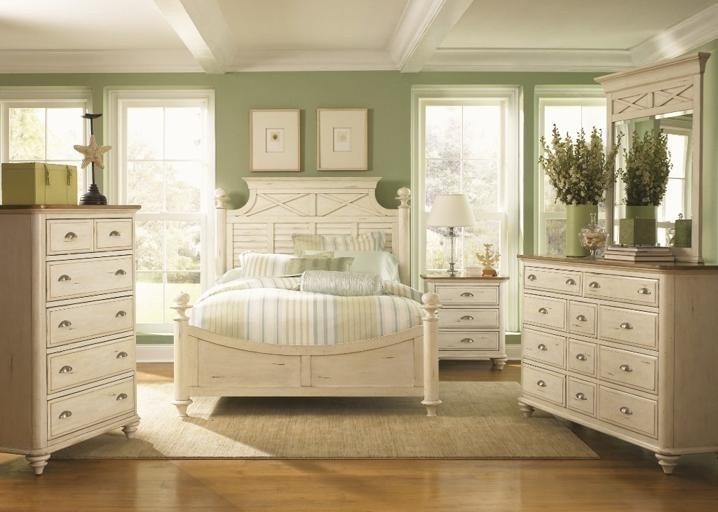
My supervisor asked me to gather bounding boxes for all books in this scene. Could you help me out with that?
[603,244,674,266]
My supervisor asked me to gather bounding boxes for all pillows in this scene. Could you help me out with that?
[240,251,399,296]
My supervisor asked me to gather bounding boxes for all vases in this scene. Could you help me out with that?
[589,246,605,261]
[626,204,655,218]
[566,204,598,256]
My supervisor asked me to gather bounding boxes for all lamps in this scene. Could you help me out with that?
[428,192,476,272]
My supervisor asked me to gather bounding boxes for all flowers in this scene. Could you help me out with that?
[621,128,672,206]
[580,224,608,249]
[535,124,625,205]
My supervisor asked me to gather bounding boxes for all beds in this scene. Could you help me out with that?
[170,177,442,420]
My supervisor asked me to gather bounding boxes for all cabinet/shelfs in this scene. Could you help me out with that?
[518,255,718,474]
[0,205,141,475]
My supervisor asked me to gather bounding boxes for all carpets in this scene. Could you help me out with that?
[25,381,601,460]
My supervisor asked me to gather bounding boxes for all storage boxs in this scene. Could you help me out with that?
[2,163,77,205]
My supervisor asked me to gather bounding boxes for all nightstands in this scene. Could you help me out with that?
[420,269,510,371]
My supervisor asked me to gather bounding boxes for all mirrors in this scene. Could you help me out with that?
[594,52,711,263]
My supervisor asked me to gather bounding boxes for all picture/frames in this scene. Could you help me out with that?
[250,109,300,171]
[317,108,368,171]
